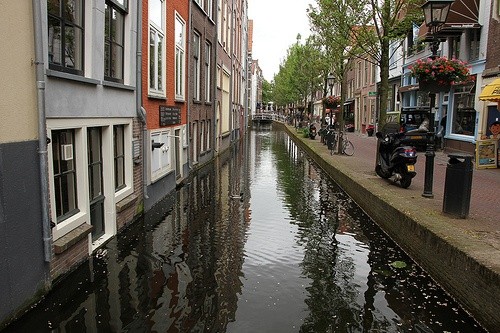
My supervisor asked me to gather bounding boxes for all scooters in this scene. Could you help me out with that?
[308,122,317,139]
[375,131,418,189]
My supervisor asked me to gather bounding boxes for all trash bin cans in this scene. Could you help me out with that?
[327,125,337,150]
[442,153,475,219]
[288,116,291,123]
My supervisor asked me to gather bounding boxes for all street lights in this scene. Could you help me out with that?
[327,72,337,149]
[422,0,454,197]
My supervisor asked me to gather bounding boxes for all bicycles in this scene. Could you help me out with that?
[321,124,355,156]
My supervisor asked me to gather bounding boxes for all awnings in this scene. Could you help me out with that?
[478,78,500,101]
